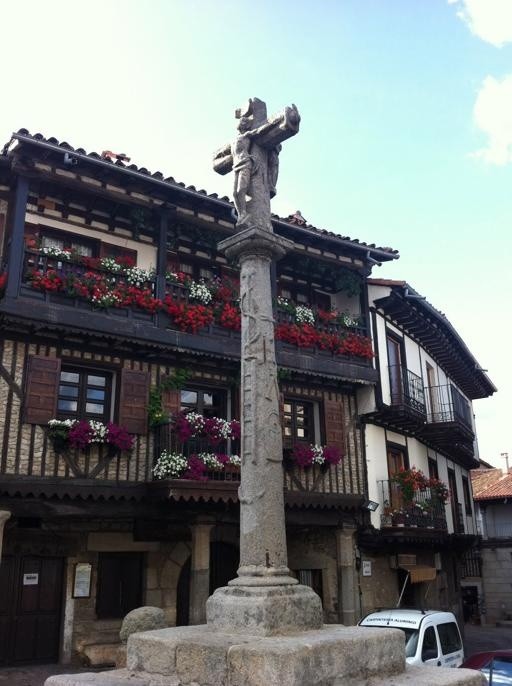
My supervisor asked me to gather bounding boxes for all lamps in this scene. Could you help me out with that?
[362,499,379,511]
[14,515,42,531]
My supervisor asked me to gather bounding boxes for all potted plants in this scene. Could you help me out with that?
[380,500,392,528]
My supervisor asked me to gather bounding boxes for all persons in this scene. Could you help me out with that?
[212,114,285,225]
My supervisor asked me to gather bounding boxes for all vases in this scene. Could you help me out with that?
[397,524,404,527]
[410,525,418,528]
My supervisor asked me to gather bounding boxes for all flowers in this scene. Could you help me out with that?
[390,465,449,524]
[151,411,343,481]
[23,232,378,359]
[47,418,134,451]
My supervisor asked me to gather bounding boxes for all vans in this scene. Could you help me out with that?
[355,606,467,669]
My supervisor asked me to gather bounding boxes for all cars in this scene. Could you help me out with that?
[458,648,512,686]
